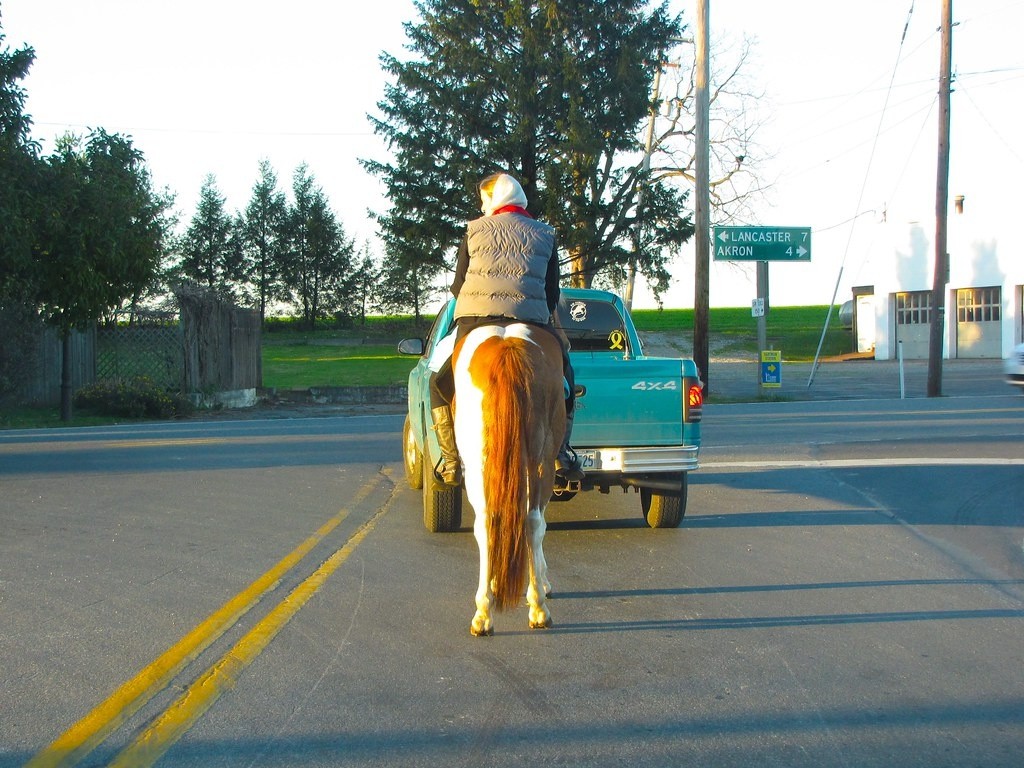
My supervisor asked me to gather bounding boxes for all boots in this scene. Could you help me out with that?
[430,405,464,486]
[554,418,585,482]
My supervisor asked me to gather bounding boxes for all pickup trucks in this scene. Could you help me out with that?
[394,287,705,533]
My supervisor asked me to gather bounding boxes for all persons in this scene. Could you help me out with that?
[428,173,585,492]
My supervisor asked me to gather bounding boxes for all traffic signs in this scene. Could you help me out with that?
[712,226,813,262]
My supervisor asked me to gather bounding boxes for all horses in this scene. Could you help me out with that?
[447,319,567,638]
